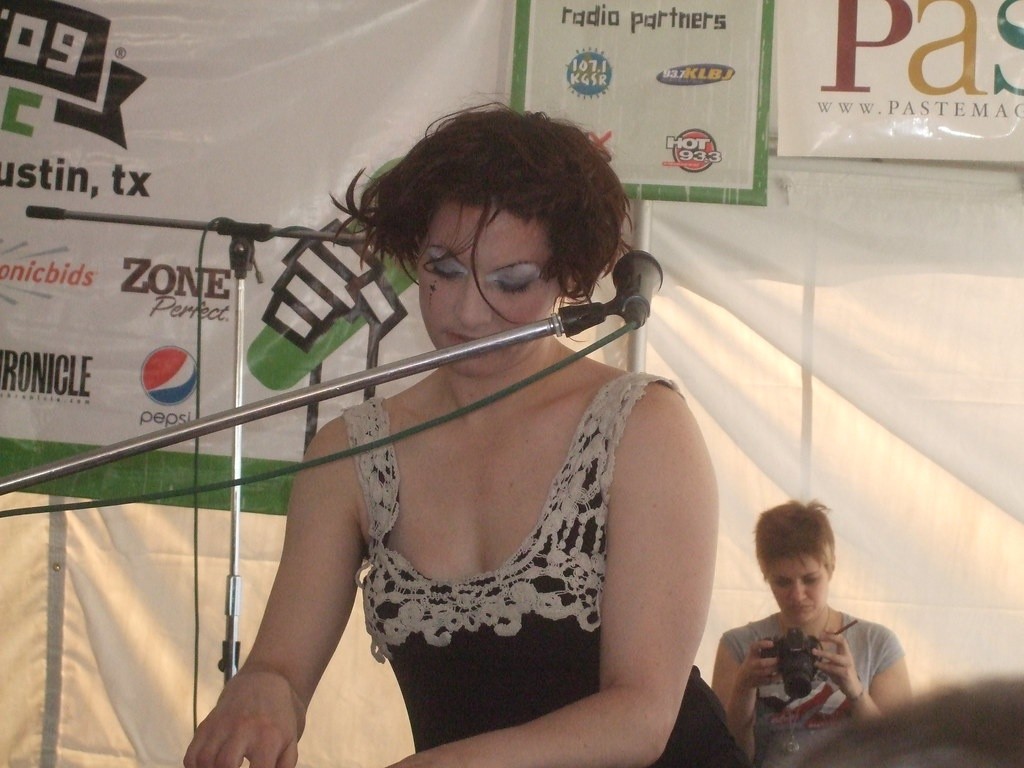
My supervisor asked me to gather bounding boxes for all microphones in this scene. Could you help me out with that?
[613,250,663,330]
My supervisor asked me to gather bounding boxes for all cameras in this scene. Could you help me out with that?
[761,627,824,698]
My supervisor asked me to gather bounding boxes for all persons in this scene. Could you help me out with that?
[712,499,910,767]
[182,101,719,768]
